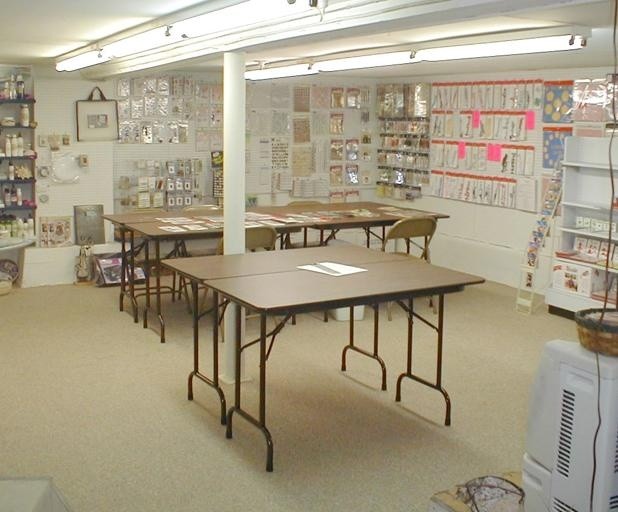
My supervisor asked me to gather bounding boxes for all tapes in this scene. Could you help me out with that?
[40,194,48,203]
[39,167,49,178]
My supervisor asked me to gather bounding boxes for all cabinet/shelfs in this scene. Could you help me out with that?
[545,136,618,320]
[0,98,39,251]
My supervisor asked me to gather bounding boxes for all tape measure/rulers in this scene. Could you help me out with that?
[314,263,340,274]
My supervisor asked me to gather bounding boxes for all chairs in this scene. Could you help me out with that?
[198,225,276,344]
[380,214,438,321]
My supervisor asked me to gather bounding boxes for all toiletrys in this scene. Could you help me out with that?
[8,160,15,180]
[5,132,23,156]
[0,216,34,241]
[4,73,25,99]
[4,183,22,206]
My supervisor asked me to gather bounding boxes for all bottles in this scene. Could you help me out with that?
[2,117,15,126]
[0,73,26,99]
[19,104,31,127]
[9,162,15,180]
[4,183,23,207]
[6,132,23,157]
[0,213,34,240]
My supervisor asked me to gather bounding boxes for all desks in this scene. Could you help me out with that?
[203,258,485,473]
[159,243,412,425]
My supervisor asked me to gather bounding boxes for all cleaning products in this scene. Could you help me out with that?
[0,71,36,243]
